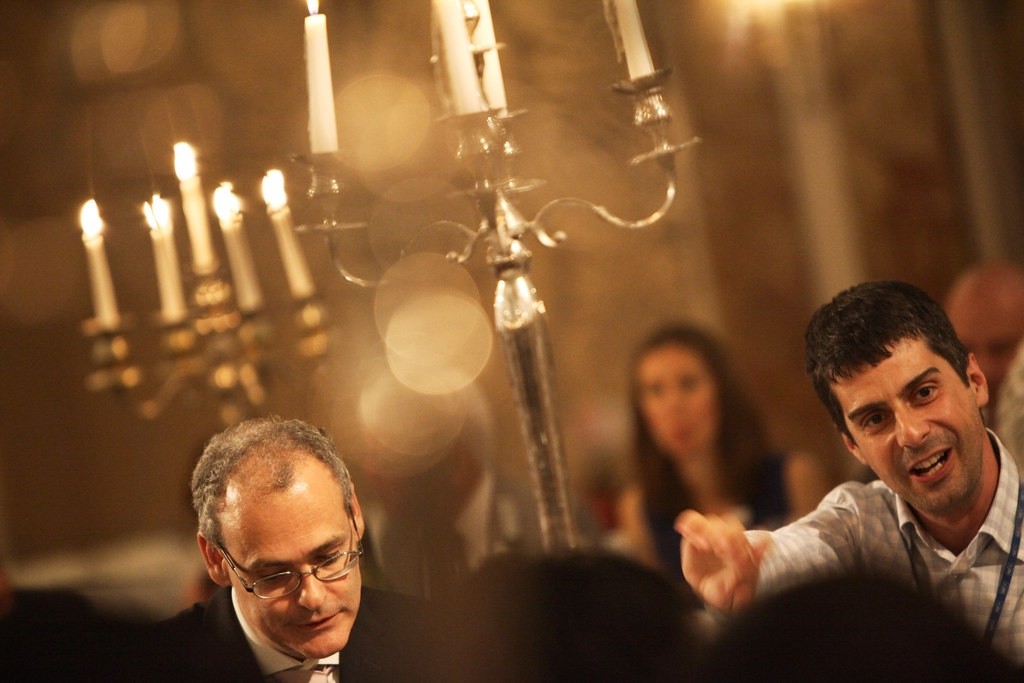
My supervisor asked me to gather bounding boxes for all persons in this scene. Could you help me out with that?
[624,318,830,597]
[140,416,486,683]
[681,279,1022,672]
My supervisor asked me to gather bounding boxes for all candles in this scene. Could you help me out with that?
[602,2,655,81]
[301,0,344,154]
[172,141,221,277]
[211,182,265,316]
[261,168,318,301]
[430,0,508,122]
[142,193,186,324]
[78,197,123,331]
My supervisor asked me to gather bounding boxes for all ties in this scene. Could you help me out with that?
[271,664,335,683]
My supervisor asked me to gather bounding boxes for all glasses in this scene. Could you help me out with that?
[217,502,363,598]
